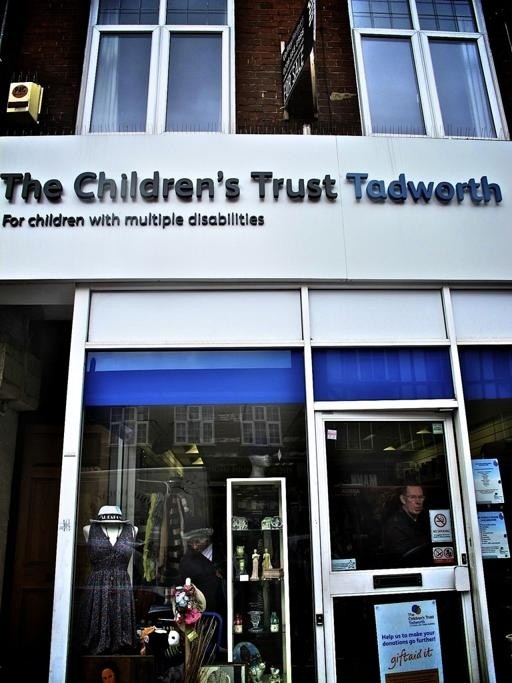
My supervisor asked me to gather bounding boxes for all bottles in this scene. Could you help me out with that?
[233,612,243,634]
[270,610,280,633]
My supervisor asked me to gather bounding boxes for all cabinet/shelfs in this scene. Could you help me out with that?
[226,477,292,683]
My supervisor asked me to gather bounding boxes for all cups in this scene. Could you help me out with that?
[248,610,264,631]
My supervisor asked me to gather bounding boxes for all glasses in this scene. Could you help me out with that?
[404,495,426,501]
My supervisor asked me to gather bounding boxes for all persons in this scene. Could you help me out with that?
[383,482,455,570]
[95,660,123,682]
[245,454,273,477]
[82,522,140,655]
[252,548,261,579]
[262,548,274,581]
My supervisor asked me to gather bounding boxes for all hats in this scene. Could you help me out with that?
[90,505,130,523]
[183,518,212,540]
